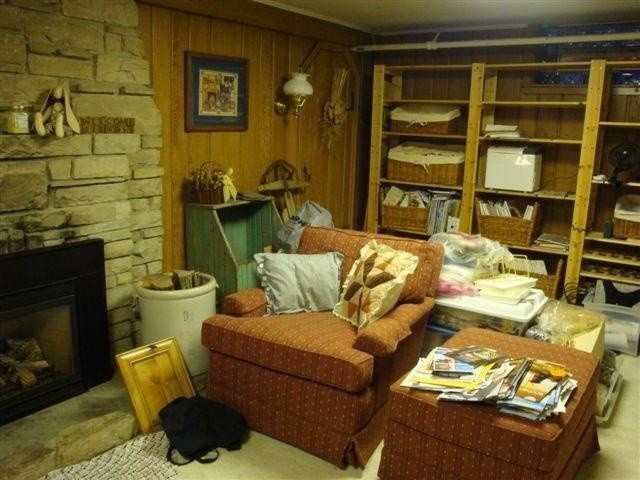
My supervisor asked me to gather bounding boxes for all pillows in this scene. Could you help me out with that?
[253,252,344,316]
[332,238,421,334]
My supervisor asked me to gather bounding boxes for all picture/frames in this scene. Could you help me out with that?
[184,50,248,132]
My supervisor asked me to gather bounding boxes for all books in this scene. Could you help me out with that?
[483,124,518,132]
[479,288,530,305]
[534,232,568,252]
[475,196,533,220]
[473,271,539,291]
[485,132,522,138]
[382,184,462,236]
[399,344,579,423]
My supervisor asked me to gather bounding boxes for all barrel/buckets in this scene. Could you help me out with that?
[136,271,216,378]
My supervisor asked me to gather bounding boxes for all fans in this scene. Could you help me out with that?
[606,144,639,183]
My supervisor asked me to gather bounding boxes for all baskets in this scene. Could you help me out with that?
[610,193,640,240]
[473,196,545,247]
[379,102,467,232]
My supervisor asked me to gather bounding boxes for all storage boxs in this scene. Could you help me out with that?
[421,288,549,359]
[485,145,544,192]
[582,289,640,357]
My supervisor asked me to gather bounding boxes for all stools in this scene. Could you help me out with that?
[377,326,601,479]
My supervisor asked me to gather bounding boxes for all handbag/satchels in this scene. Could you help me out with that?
[158,394,249,465]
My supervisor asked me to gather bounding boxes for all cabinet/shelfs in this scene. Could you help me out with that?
[366,59,640,304]
[184,192,284,313]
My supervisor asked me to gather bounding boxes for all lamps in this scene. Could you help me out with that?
[274,65,314,118]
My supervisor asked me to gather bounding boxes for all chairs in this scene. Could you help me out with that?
[202,226,444,467]
